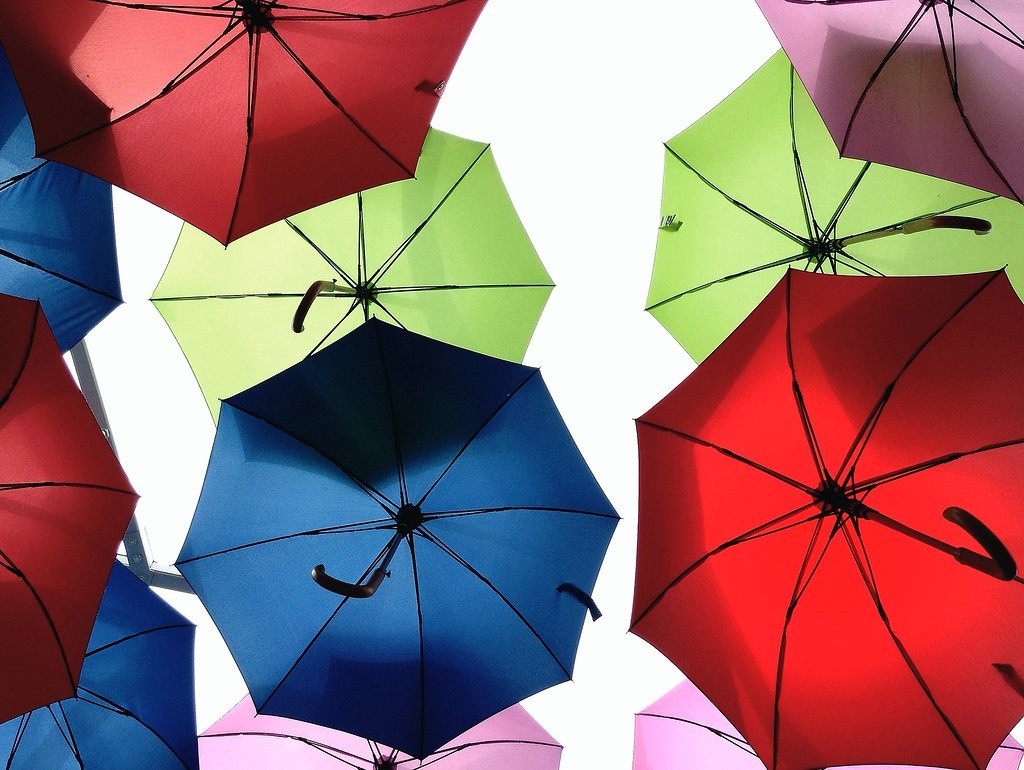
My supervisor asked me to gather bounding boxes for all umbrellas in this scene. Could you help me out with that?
[642,45,1022,368]
[622,271,1024,768]
[755,0,1020,203]
[0,0,616,770]
